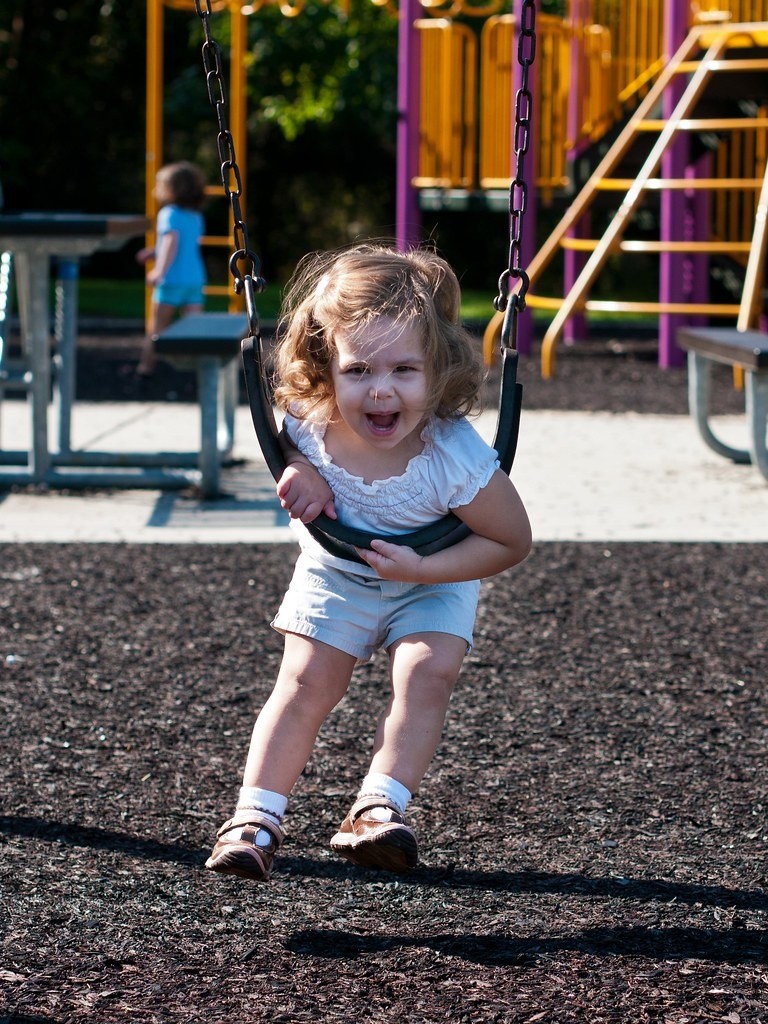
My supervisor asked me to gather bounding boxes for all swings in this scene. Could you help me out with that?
[190,1,536,562]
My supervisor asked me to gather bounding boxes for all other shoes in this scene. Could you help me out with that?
[115,366,150,391]
[204,814,284,876]
[329,793,419,866]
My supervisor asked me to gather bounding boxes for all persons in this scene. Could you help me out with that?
[199,244,534,868]
[139,162,205,375]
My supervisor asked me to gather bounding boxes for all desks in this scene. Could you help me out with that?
[0,212,225,491]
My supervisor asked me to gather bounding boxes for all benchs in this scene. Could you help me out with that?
[674,324,768,488]
[151,310,255,503]
